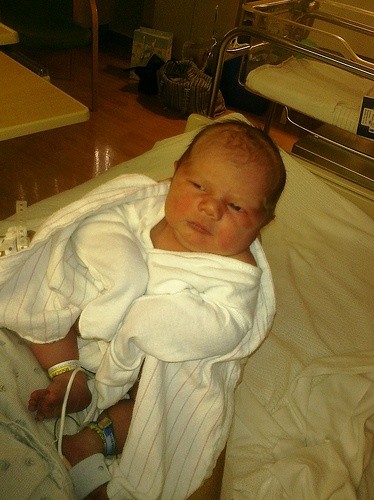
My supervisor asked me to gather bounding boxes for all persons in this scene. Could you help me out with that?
[25,120,287,500]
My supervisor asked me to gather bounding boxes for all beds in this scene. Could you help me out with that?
[238,0,374,161]
[0,114,374,500]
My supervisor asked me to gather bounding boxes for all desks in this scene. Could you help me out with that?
[0,51,91,145]
